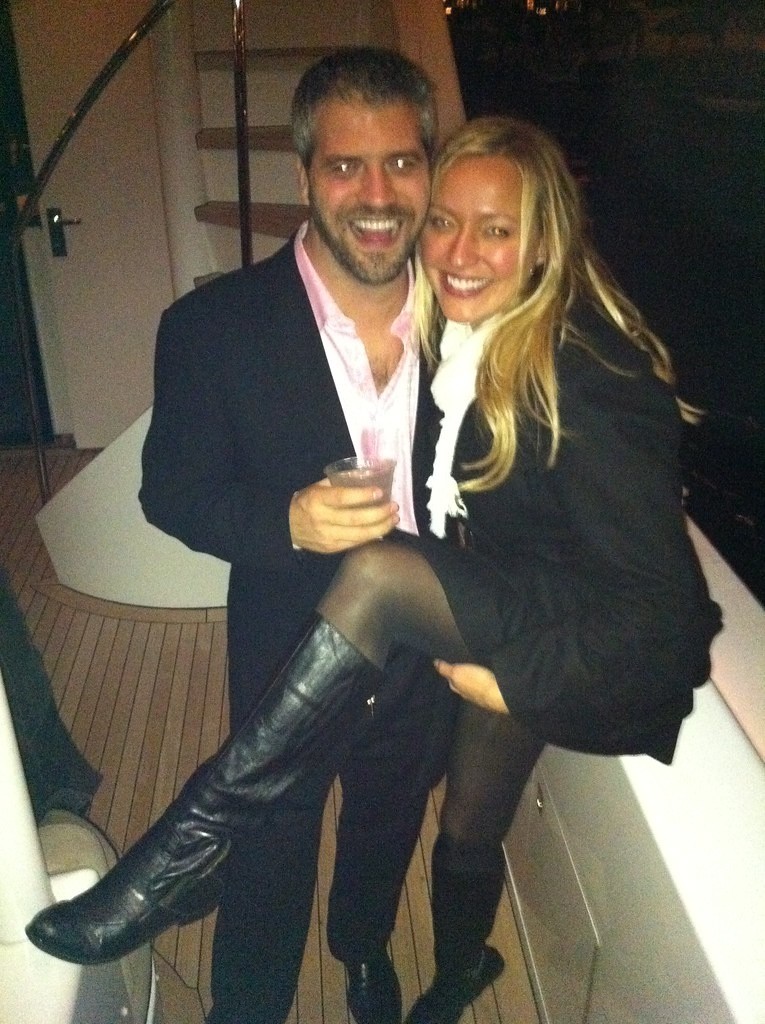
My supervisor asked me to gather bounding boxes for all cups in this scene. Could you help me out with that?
[324,456,398,542]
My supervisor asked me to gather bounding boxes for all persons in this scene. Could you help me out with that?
[1,560,103,830]
[26,116,724,1024]
[138,46,457,1024]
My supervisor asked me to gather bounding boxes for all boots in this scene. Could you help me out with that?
[21,606,386,964]
[396,840,508,1023]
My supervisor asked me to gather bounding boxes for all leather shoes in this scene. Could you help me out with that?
[337,959,401,1024]
